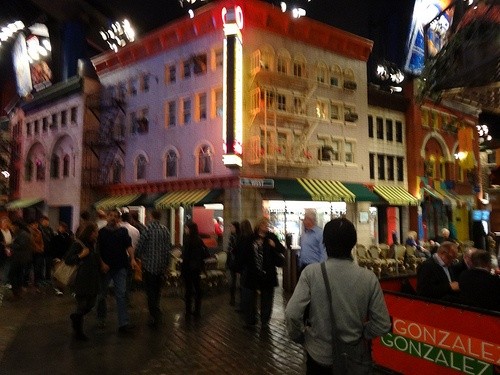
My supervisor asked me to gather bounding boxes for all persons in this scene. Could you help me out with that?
[0,206,500,375]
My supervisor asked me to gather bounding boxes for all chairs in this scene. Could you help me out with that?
[357,242,431,280]
[168,248,226,296]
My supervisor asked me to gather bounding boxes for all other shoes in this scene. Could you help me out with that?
[118,323,136,331]
[96,320,108,328]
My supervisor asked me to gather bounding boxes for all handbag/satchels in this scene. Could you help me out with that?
[52,239,88,288]
[332,335,374,375]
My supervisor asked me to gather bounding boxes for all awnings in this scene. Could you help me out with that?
[423,187,466,206]
[258,176,379,203]
[8,198,43,208]
[91,188,222,209]
[373,185,420,206]
